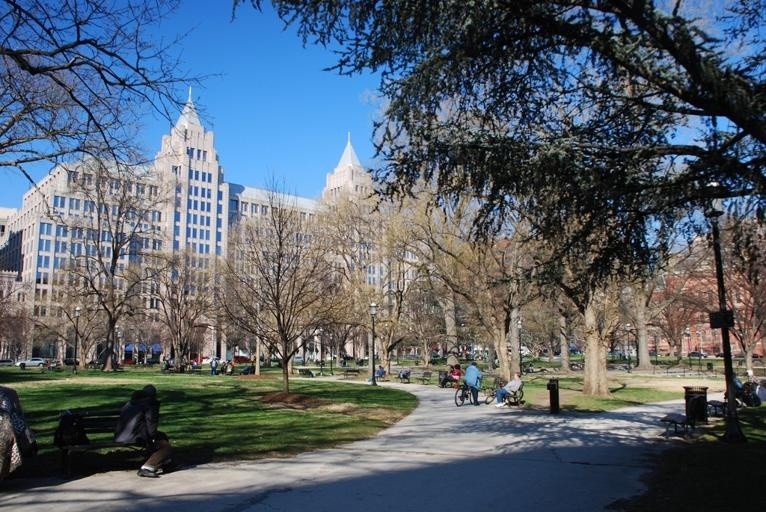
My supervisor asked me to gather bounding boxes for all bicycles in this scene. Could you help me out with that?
[455,381,474,406]
[485,388,523,405]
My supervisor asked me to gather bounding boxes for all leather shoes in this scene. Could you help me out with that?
[137,468,166,478]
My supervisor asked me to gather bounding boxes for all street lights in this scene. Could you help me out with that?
[517,320,524,376]
[73,307,80,374]
[114,325,118,371]
[369,303,379,385]
[703,182,748,444]
[625,323,632,373]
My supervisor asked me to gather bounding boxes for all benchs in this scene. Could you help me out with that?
[660,392,728,440]
[416,372,431,384]
[21,404,138,479]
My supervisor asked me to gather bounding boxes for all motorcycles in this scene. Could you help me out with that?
[734,373,761,408]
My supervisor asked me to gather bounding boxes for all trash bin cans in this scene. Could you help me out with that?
[683,386,708,424]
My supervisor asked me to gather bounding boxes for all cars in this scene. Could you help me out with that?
[17,358,47,369]
[0,359,14,367]
[688,351,707,357]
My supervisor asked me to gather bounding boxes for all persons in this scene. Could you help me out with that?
[113,383,170,477]
[374,364,385,376]
[439,364,462,387]
[210,358,218,375]
[496,372,522,406]
[465,362,483,405]
[746,369,760,386]
[724,371,744,398]
[160,355,199,374]
[449,366,455,374]
[220,361,227,375]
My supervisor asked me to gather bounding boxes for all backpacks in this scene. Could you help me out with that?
[54,408,92,448]
